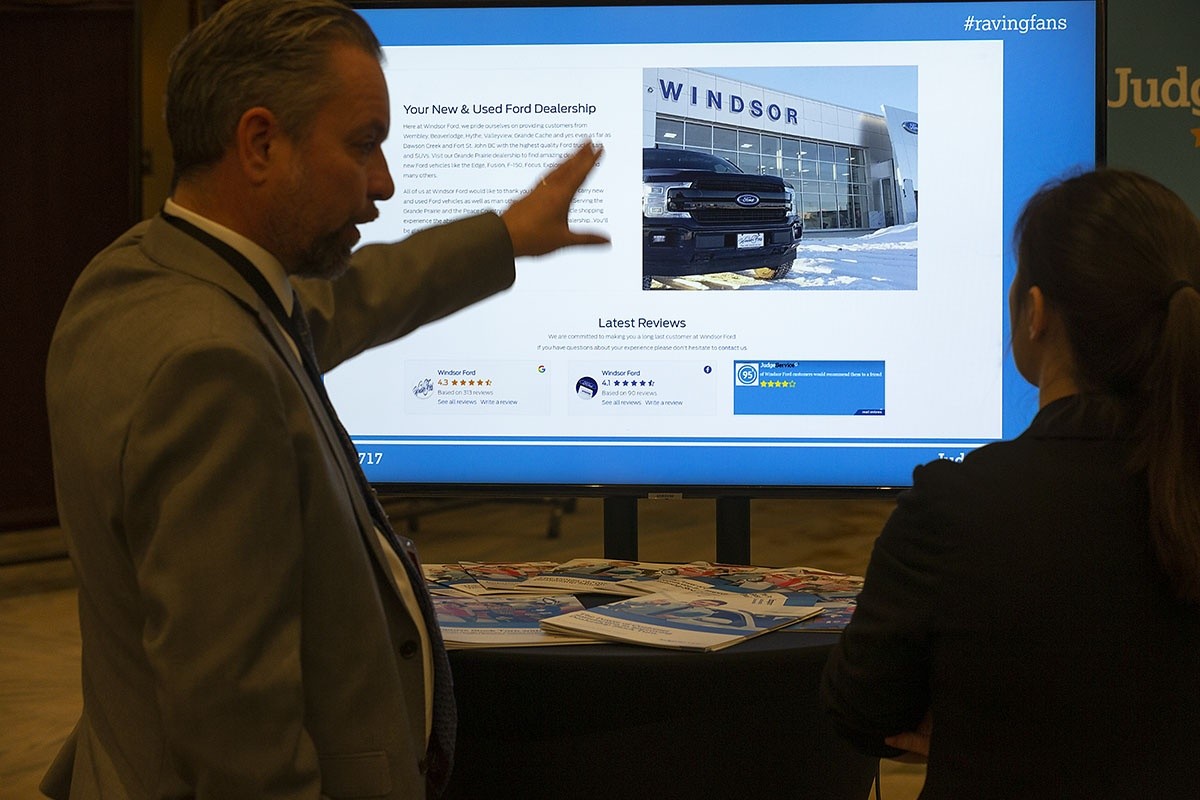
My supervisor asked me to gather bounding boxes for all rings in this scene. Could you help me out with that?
[542,180,547,186]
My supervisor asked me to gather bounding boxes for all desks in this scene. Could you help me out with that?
[420,559,864,800]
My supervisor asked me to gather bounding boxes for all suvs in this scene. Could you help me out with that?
[641,146,804,293]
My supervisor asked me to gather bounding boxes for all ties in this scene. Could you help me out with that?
[291,289,456,795]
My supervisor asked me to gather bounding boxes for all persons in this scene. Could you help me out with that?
[619,598,727,621]
[824,169,1200,800]
[41,0,611,800]
